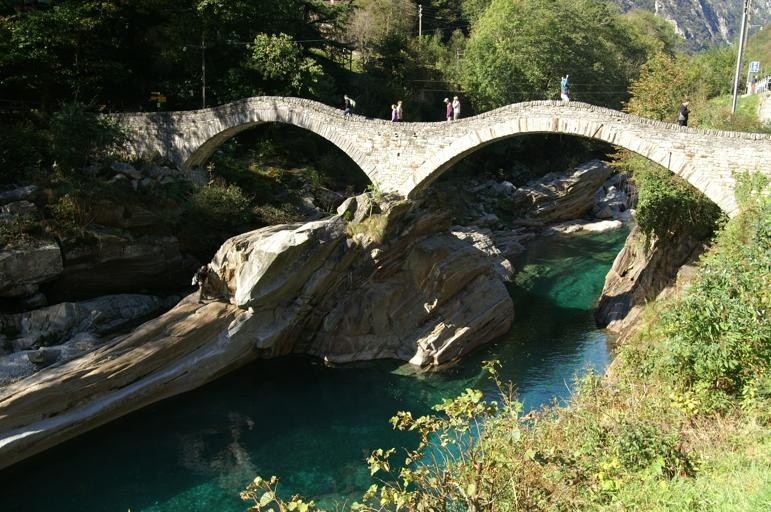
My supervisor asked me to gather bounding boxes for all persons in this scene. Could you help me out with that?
[196,265,212,304]
[398,100,404,122]
[344,95,354,117]
[443,98,454,120]
[679,99,692,126]
[562,75,571,102]
[452,96,461,120]
[391,104,398,122]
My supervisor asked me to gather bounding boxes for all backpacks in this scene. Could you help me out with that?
[349,98,355,108]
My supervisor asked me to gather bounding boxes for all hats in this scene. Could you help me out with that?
[443,96,458,102]
